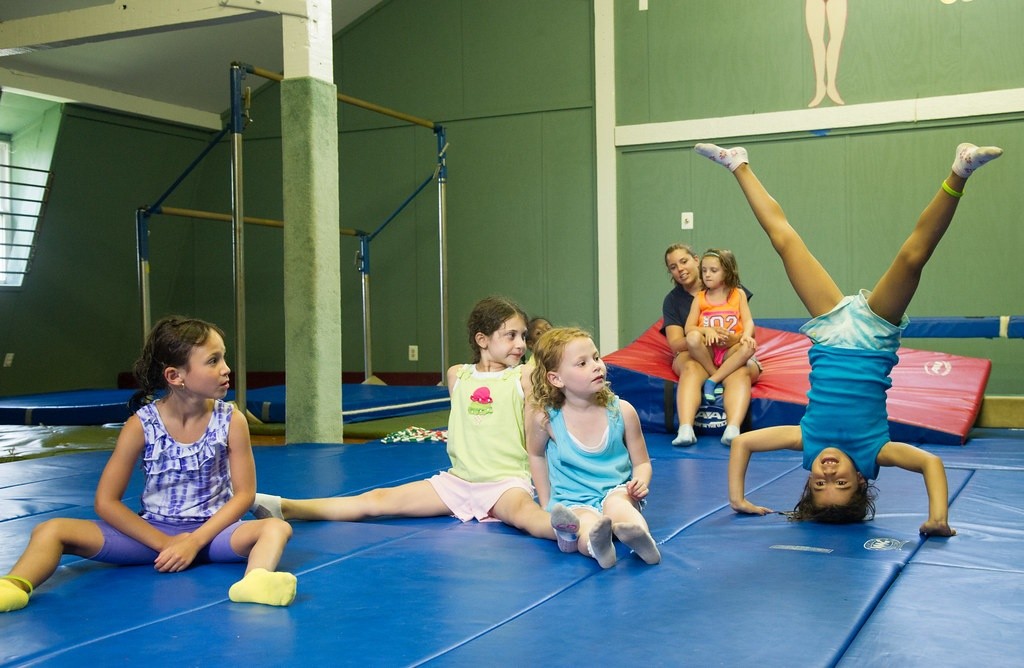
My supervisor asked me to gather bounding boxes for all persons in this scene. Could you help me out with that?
[659,243,763,446]
[1,313,298,612]
[694,139,998,537]
[525,316,556,366]
[527,327,661,570]
[248,294,581,553]
